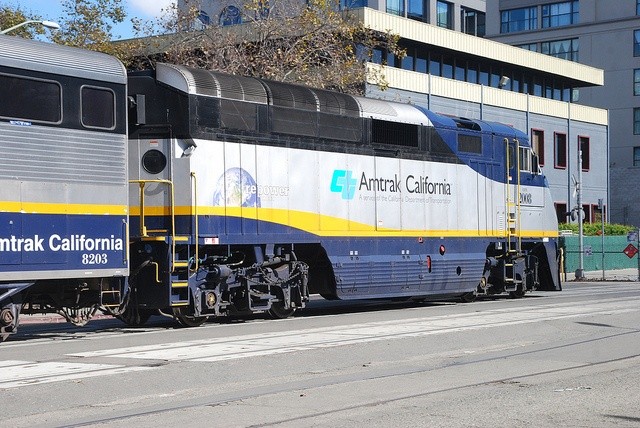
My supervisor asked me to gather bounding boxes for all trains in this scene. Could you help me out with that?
[0,33,565,342]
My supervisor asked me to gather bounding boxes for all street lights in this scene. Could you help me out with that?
[1,20,60,34]
